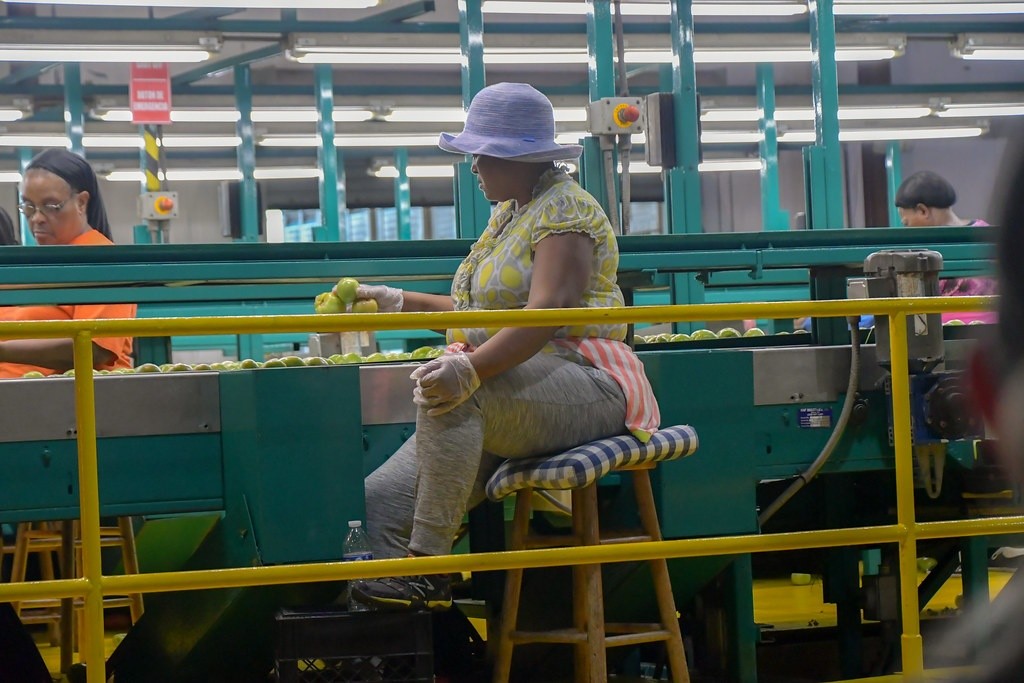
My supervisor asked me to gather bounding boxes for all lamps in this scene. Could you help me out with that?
[0,89,1024,184]
[949,32,1024,61]
[283,32,909,64]
[0,29,224,63]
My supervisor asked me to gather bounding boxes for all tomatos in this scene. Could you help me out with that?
[632,328,765,343]
[941,319,985,327]
[20,278,443,379]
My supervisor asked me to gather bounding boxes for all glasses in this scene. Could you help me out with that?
[17,191,77,215]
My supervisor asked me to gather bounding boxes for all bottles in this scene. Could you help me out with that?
[343,520,377,612]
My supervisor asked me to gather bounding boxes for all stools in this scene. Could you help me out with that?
[10,516,145,674]
[492,460,690,683]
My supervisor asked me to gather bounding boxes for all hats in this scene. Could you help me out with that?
[438,81,581,164]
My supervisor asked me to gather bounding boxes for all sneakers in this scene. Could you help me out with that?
[351,552,453,612]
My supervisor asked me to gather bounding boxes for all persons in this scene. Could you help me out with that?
[0,147,136,381]
[895,171,998,324]
[930,130,1024,683]
[331,83,661,611]
